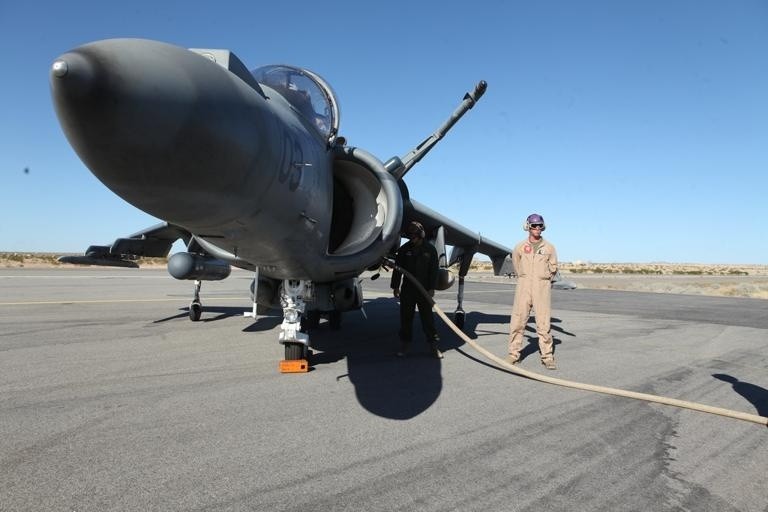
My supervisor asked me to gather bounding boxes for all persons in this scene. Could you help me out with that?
[388,221,439,359]
[507,213,556,370]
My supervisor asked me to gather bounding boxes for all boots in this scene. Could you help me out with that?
[396,341,406,356]
[430,344,442,358]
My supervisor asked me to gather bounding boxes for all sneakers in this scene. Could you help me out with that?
[503,356,520,364]
[544,361,555,369]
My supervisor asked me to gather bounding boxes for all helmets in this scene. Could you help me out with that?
[407,221,425,239]
[523,214,545,231]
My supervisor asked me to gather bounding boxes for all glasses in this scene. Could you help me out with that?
[529,222,544,229]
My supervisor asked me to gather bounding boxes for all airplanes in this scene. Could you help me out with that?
[45,33,573,394]
